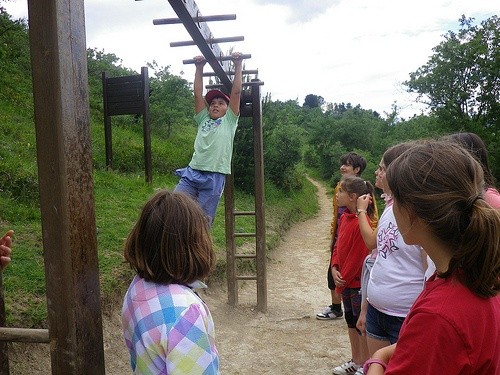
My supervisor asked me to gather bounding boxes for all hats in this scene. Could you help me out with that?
[205,90,231,105]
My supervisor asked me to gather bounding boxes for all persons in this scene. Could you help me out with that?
[356,143,436,357]
[331,176,375,375]
[120,190,220,375]
[448,133,500,210]
[172,52,243,231]
[316,152,379,320]
[366,141,500,375]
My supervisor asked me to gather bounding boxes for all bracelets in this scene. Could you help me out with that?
[363,359,387,375]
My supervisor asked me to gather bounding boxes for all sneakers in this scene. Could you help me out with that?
[332,360,359,375]
[354,367,365,375]
[317,307,344,320]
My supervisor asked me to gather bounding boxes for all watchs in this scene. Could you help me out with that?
[356,209,367,215]
